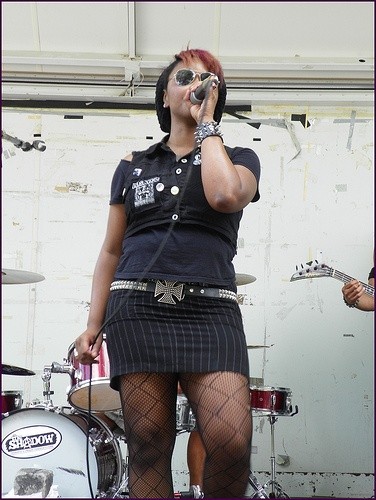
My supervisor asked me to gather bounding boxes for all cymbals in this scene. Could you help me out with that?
[2,363,36,376]
[235,273,257,286]
[2,268,45,285]
[246,344,270,349]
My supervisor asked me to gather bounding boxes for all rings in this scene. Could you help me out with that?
[74,348,77,356]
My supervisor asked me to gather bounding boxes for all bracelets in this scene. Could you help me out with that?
[194,120,224,155]
[342,296,359,308]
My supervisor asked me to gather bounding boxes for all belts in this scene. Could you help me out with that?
[109,279,239,304]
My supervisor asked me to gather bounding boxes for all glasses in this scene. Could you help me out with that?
[166,68,219,89]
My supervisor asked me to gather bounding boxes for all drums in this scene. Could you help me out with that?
[176,396,196,431]
[66,332,122,411]
[2,406,123,498]
[249,386,291,414]
[1,390,23,414]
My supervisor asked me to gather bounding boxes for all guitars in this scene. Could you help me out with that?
[290,259,374,297]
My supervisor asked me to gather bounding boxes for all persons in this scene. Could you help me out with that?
[187,425,207,492]
[72,41,260,500]
[342,267,375,312]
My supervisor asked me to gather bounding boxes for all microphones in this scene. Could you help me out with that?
[189,73,212,105]
